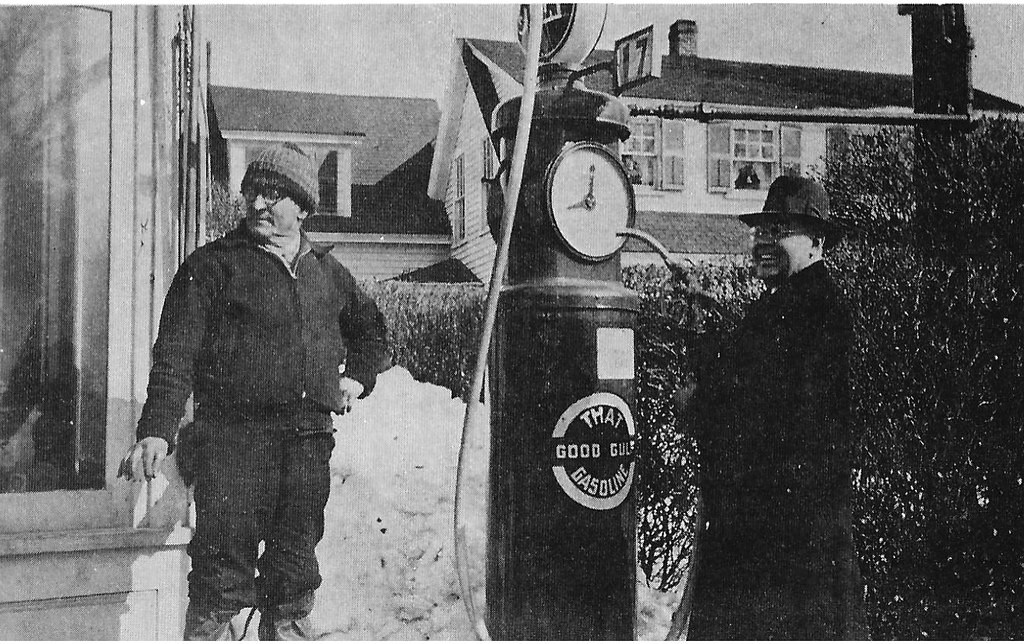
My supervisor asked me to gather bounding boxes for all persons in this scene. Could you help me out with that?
[677,173,870,641]
[117,144,386,641]
[622,155,643,183]
[736,164,761,189]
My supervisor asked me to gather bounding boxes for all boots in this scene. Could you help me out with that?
[258,617,316,641]
[184,601,236,641]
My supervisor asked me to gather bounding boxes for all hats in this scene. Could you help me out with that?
[738,175,852,248]
[240,143,319,214]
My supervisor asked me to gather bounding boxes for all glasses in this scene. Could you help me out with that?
[240,184,288,202]
[754,222,809,242]
